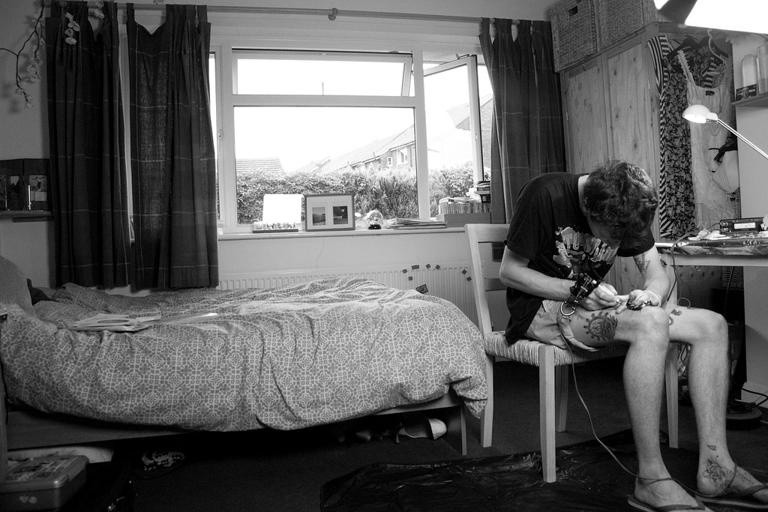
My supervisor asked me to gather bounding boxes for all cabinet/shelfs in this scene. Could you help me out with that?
[545,0,671,74]
[560,22,744,319]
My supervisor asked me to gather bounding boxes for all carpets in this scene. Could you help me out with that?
[318,426,768,512]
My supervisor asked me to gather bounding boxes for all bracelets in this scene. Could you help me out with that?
[644,289,661,306]
[568,282,589,305]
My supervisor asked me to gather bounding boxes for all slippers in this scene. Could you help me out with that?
[695,484,768,509]
[628,493,713,511]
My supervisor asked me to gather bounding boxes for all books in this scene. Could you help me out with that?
[72,306,162,335]
[385,215,447,232]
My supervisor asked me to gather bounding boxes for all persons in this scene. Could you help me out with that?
[497,158,766,510]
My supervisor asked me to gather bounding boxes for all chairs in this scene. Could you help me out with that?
[463,222,681,486]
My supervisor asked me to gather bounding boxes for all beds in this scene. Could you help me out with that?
[0,256,487,461]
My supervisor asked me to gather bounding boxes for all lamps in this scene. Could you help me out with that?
[682,104,768,161]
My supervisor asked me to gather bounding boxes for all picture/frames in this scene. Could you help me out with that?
[304,193,356,232]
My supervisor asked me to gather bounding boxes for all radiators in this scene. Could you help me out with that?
[212,264,494,337]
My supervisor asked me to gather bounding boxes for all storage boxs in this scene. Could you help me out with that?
[0,455,87,511]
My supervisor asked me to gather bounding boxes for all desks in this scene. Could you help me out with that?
[659,251,768,268]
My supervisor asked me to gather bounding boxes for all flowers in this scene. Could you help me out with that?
[0,0,114,120]
